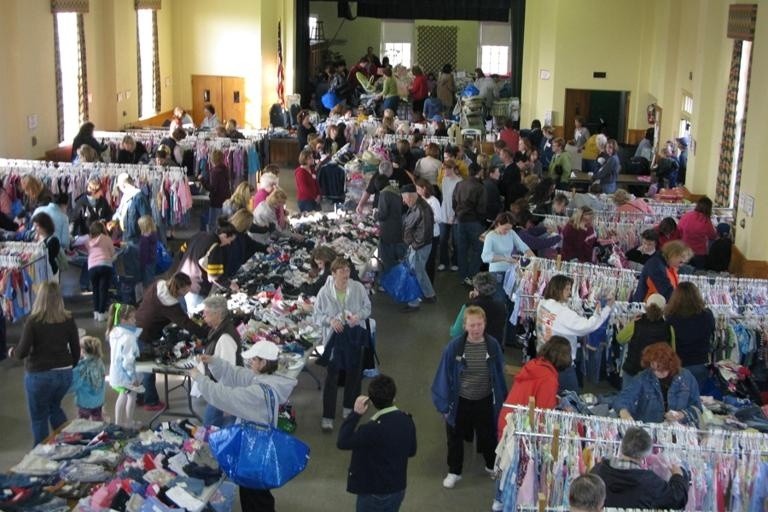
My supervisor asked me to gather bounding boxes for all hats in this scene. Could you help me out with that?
[645,293,666,309]
[241,340,280,361]
[715,223,730,235]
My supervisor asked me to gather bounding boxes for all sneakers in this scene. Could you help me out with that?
[424,297,438,305]
[144,400,162,410]
[444,473,459,489]
[400,305,420,312]
[128,421,143,430]
[93,311,107,322]
[320,417,333,431]
[438,263,459,271]
[343,408,352,418]
[492,499,503,512]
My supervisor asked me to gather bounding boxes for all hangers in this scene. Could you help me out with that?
[516,402,768,487]
[107,129,261,153]
[543,189,734,239]
[0,155,188,186]
[517,256,767,327]
[358,116,452,144]
[0,240,46,269]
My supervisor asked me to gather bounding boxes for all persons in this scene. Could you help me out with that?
[0,47,731,512]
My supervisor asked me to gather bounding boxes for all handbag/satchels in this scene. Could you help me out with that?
[381,260,421,303]
[206,423,310,489]
[197,256,208,270]
[622,156,651,175]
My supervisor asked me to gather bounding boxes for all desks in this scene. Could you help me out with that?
[0,418,227,512]
[149,204,382,427]
